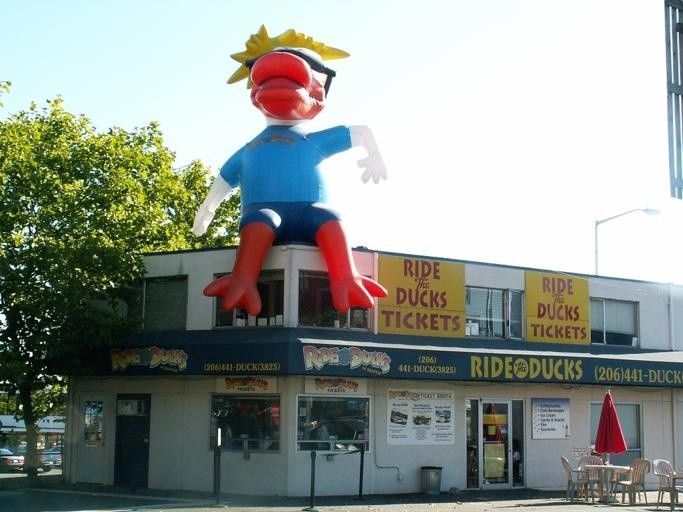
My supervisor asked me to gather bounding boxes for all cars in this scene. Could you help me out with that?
[300,418,370,451]
[0,448,25,473]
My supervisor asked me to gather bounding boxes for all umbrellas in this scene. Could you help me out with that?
[595,388,627,503]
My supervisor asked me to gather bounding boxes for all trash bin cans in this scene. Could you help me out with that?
[421,466,442,496]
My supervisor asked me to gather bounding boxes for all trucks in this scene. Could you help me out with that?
[3,441,62,473]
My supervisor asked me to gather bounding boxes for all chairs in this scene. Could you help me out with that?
[559,455,683,512]
